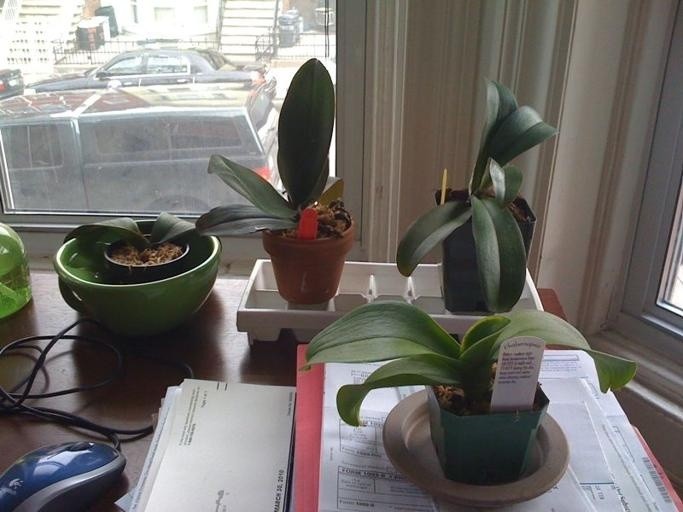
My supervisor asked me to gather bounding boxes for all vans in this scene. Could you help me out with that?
[0,88,285,221]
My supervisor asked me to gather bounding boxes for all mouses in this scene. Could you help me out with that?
[0,437,126,512]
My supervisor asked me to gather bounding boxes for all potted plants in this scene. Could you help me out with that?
[196,58,357,305]
[395,75,561,315]
[299,299,638,505]
[67,207,201,283]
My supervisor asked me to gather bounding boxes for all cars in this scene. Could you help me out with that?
[25,50,277,133]
[4,68,30,99]
[192,45,277,104]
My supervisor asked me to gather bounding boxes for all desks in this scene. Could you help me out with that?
[2,270,683,512]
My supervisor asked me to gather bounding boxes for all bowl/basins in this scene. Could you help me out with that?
[53,220,222,330]
